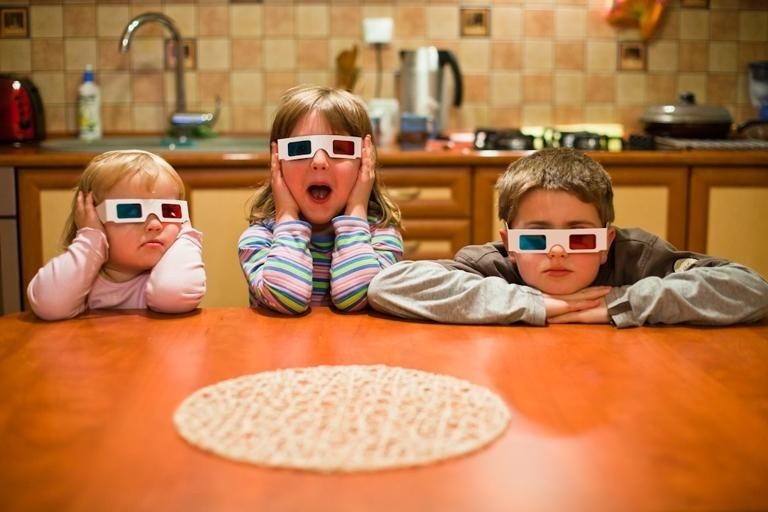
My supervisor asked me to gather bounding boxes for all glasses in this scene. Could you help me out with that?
[503,221,611,255]
[96,198,190,225]
[275,134,364,161]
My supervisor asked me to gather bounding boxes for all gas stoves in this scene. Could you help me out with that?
[474,126,658,153]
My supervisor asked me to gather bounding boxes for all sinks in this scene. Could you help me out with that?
[35,135,274,153]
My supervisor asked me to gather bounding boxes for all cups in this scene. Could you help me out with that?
[399,115,436,150]
[371,97,398,145]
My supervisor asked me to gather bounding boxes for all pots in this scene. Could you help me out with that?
[640,92,768,140]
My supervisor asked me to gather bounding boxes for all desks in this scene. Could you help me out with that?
[0,306,768,511]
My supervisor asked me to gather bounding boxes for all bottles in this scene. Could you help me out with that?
[398,46,462,139]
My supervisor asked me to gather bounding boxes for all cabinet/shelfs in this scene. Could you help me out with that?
[377,166,472,264]
[473,165,768,282]
[17,168,270,308]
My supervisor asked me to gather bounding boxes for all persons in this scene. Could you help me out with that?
[26,149,206,321]
[367,147,768,329]
[237,84,407,316]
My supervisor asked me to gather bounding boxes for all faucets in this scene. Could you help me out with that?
[119,13,185,113]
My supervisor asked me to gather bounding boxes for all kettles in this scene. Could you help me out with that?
[0,72,45,148]
